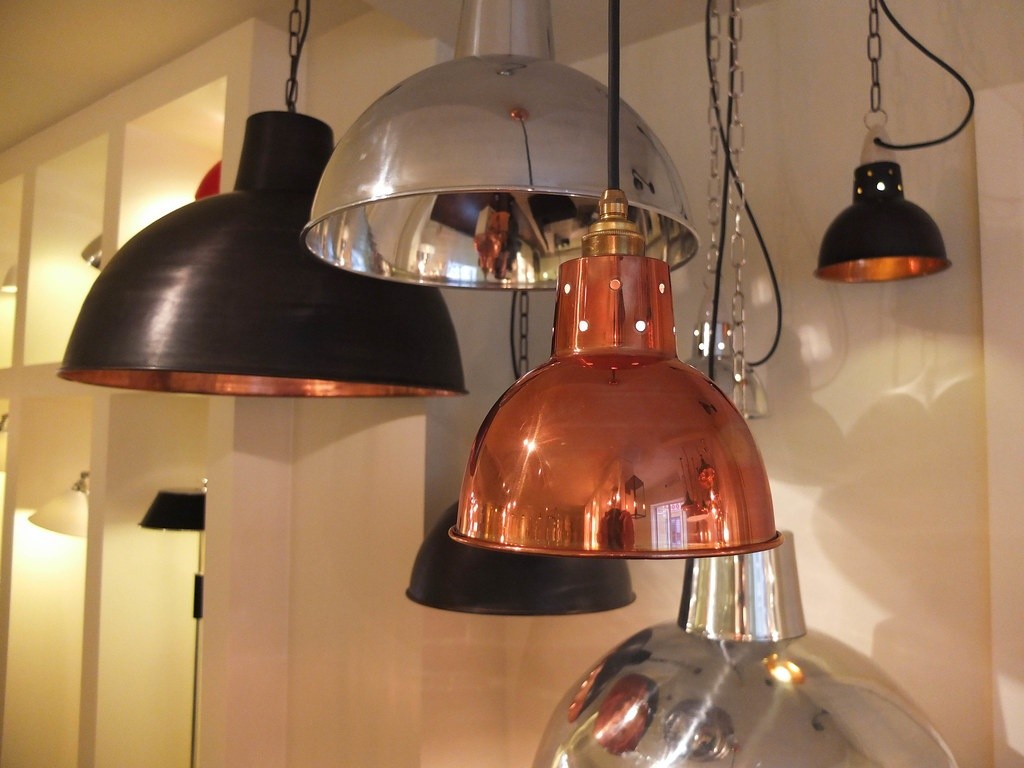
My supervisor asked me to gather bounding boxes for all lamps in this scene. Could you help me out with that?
[84,233,105,271]
[453,424,749,550]
[538,1,964,768]
[196,156,226,200]
[446,0,786,557]
[565,628,811,762]
[813,2,955,285]
[405,288,639,623]
[2,262,20,295]
[138,479,202,768]
[57,1,470,402]
[27,470,90,537]
[312,0,702,292]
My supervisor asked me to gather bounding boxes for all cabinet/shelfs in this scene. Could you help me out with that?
[3,11,288,766]
